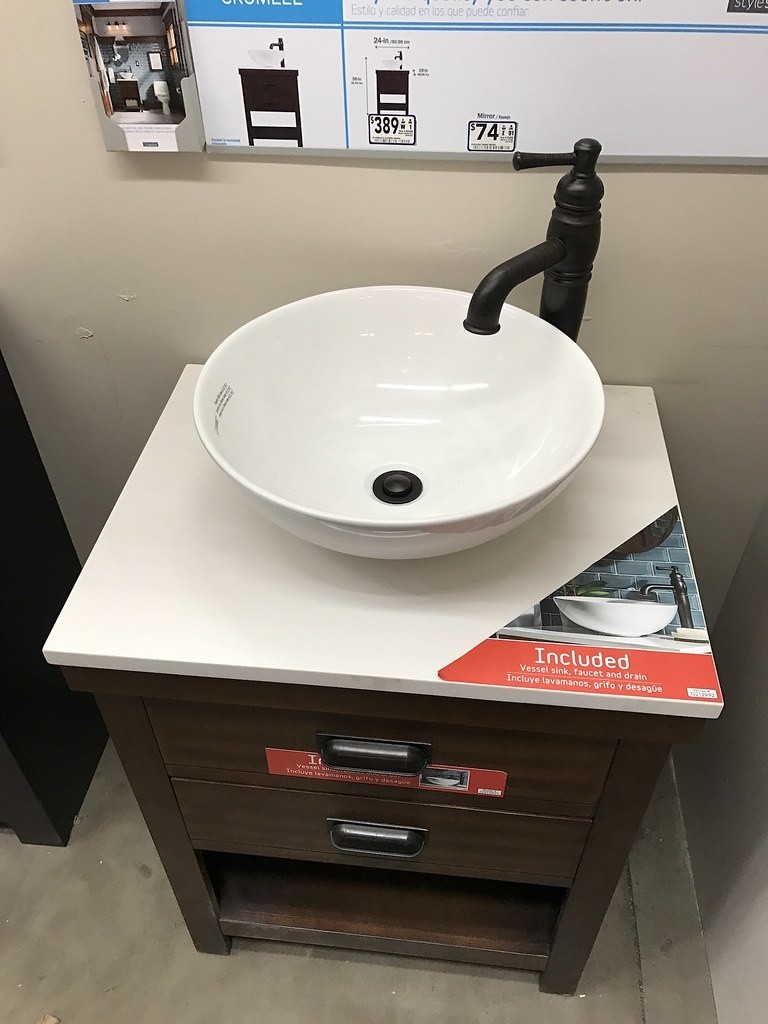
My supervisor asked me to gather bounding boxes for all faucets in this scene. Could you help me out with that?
[462,137,605,343]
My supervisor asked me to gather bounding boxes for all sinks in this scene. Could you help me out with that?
[192,285,605,559]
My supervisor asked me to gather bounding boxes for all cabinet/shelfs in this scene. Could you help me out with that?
[61,665,684,997]
[118,78,143,112]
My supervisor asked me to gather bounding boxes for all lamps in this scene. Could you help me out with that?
[113,35,126,58]
